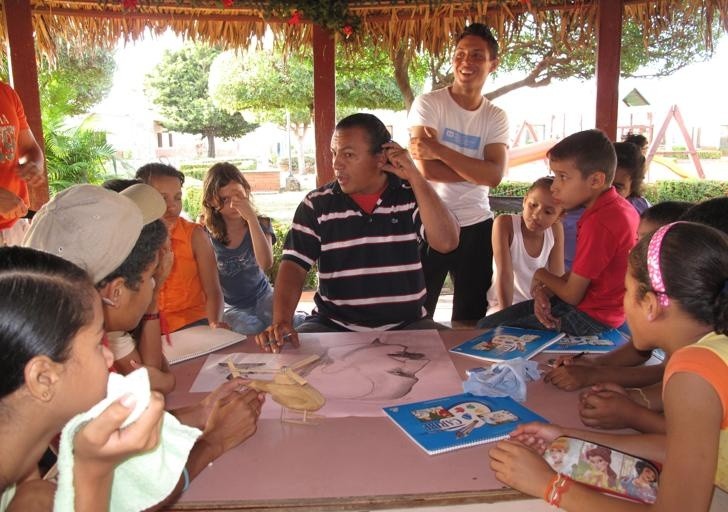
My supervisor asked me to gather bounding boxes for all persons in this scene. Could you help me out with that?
[17,183,267,512]
[408,23,510,322]
[136,164,232,334]
[0,81,46,250]
[487,221,728,512]
[679,197,728,233]
[195,162,310,335]
[100,180,176,396]
[557,134,652,275]
[476,129,643,335]
[636,201,696,243]
[1,246,166,512]
[485,175,564,314]
[255,112,461,352]
[631,387,652,411]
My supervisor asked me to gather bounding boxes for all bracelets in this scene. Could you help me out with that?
[545,473,572,506]
[141,312,159,322]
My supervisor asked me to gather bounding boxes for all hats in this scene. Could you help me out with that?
[20,181,169,291]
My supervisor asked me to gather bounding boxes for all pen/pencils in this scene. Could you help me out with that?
[558,352,585,366]
[263,333,291,347]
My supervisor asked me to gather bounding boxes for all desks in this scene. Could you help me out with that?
[162,331,637,512]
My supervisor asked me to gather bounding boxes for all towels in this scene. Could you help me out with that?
[462,356,543,404]
[50,365,205,512]
